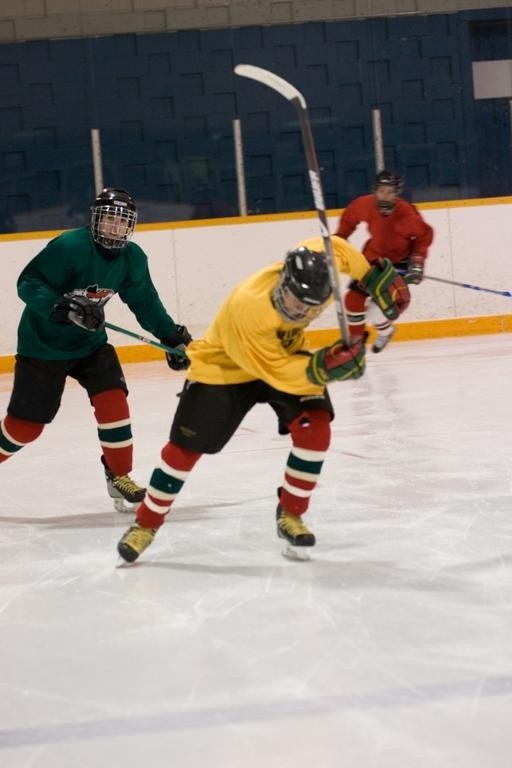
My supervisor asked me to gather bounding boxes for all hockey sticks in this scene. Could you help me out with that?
[235,64,349,351]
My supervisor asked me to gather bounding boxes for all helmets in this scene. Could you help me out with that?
[87,187,139,256]
[371,170,407,217]
[269,247,333,322]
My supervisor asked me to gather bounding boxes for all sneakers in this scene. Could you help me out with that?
[101,455,147,504]
[117,519,157,564]
[372,321,397,354]
[275,502,314,548]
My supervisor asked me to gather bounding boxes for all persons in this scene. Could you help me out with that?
[332,168,436,353]
[114,235,410,568]
[0,186,193,513]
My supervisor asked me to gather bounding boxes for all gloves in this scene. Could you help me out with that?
[354,256,410,320]
[403,252,427,286]
[161,326,194,371]
[53,293,106,332]
[307,336,365,387]
